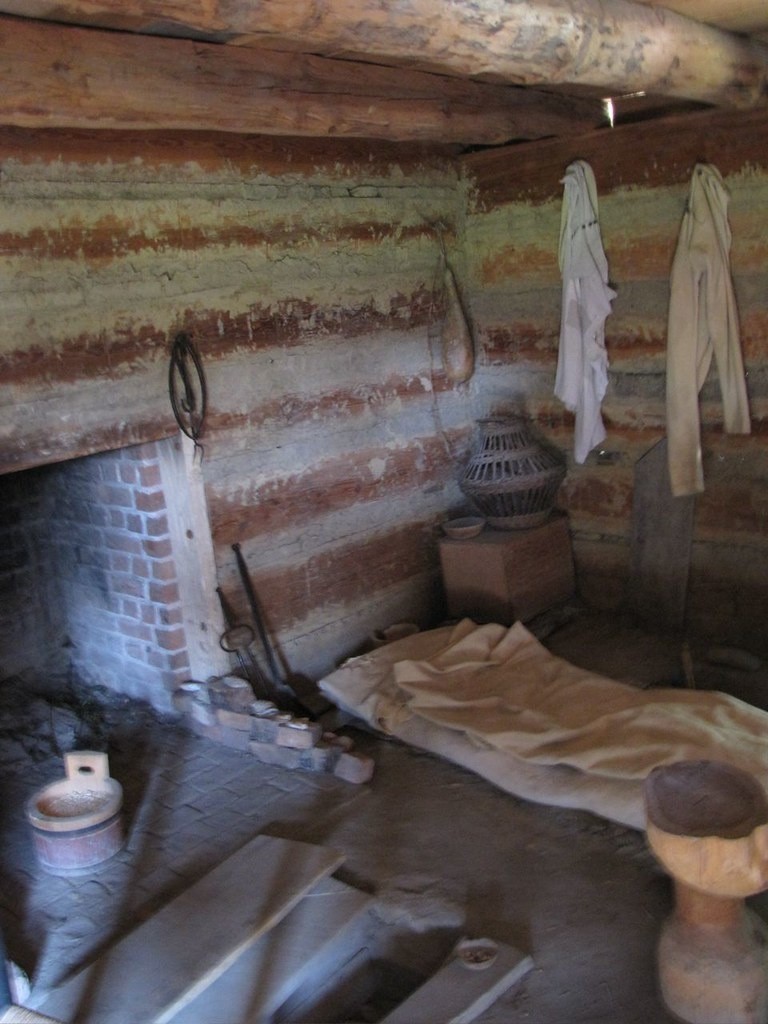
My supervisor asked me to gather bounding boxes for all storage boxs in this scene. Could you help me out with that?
[438,513,577,625]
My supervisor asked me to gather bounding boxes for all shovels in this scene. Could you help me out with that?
[231,542,316,722]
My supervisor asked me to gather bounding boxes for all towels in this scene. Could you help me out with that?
[551,157,620,466]
[667,161,751,498]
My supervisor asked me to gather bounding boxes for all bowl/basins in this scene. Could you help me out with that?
[442,517,485,540]
[456,938,497,971]
